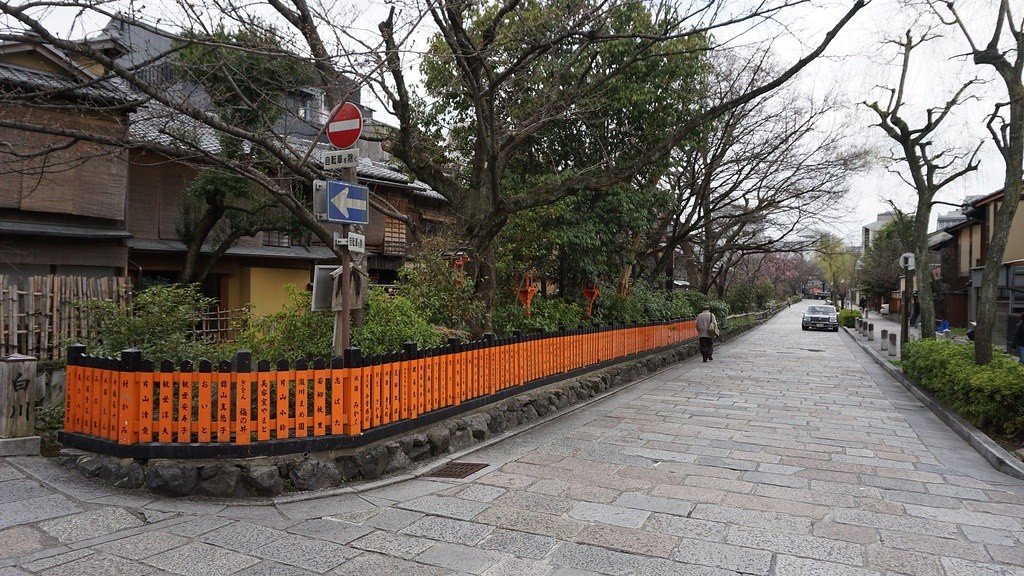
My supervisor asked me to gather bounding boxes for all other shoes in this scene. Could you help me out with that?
[703,360,707,362]
[708,355,713,361]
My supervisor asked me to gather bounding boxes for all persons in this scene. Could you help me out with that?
[696,305,718,362]
[787,296,792,308]
[860,297,867,313]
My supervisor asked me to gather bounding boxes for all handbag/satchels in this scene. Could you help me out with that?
[709,313,719,336]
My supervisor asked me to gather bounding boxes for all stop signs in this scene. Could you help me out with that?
[325,102,363,149]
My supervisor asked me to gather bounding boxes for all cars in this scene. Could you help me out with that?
[802,305,840,333]
[813,292,831,301]
